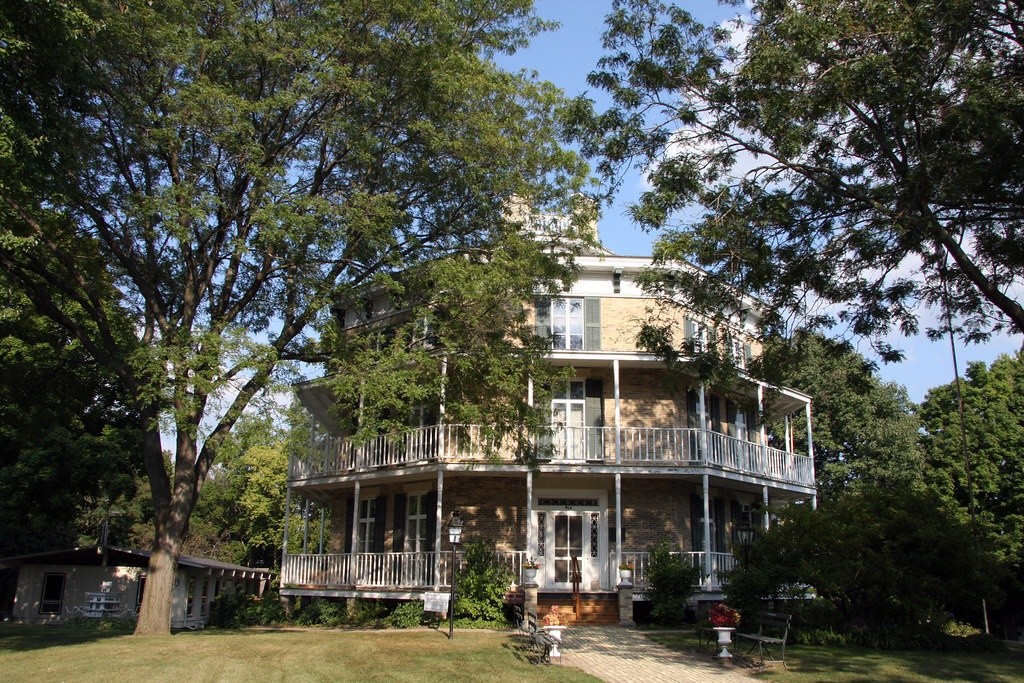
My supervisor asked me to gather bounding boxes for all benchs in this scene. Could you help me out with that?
[527,611,564,667]
[698,624,719,652]
[514,605,530,643]
[733,612,792,668]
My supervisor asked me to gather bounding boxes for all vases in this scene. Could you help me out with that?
[618,571,632,585]
[544,626,566,657]
[524,569,536,584]
[712,627,737,657]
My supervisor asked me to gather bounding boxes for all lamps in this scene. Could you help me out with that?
[175,579,180,586]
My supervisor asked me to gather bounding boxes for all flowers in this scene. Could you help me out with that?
[619,563,637,572]
[543,606,566,626]
[521,556,542,570]
[708,603,741,627]
